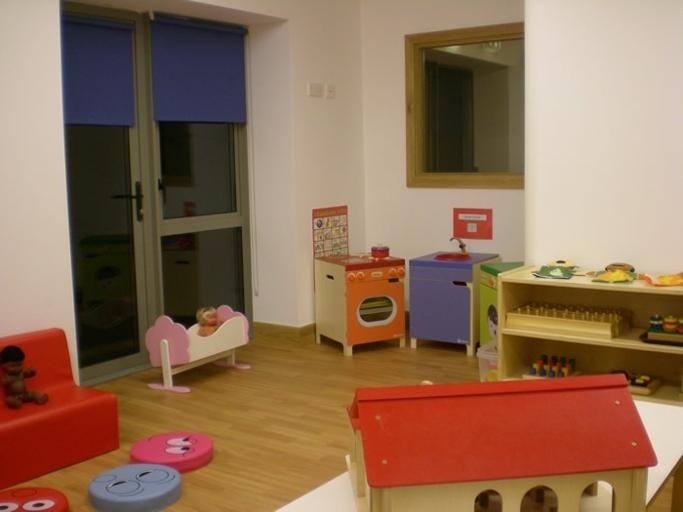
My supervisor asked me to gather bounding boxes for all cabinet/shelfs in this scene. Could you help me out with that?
[495,263,683,411]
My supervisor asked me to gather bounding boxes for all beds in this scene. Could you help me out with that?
[143,304,254,393]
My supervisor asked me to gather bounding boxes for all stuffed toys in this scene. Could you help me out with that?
[196,307,224,337]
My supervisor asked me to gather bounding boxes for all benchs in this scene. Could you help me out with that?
[0,329,119,488]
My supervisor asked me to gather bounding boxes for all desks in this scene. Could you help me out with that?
[273,400,683,511]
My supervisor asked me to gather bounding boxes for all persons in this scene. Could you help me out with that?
[0,345,48,408]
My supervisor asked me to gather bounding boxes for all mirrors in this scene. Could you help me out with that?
[403,23,524,191]
